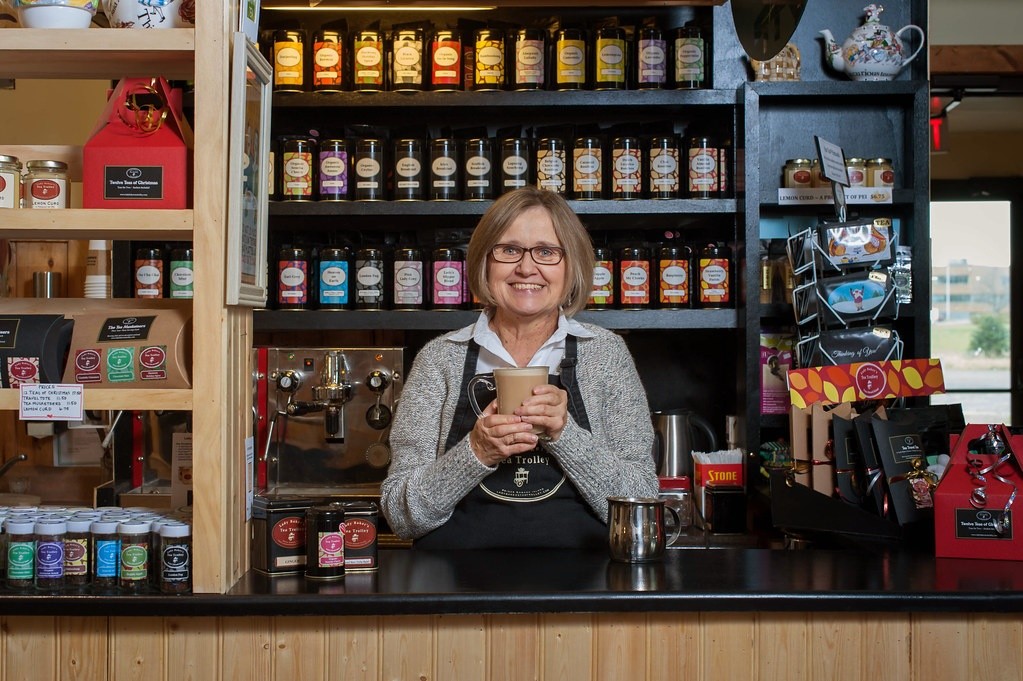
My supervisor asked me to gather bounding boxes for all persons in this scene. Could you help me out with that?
[381,186,660,555]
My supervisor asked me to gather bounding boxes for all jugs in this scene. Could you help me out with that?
[605,497,681,564]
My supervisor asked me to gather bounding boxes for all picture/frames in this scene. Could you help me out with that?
[225,30,273,307]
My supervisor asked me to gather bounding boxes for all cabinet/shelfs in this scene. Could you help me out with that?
[0,0,930,594]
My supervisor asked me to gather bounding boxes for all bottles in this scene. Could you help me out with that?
[657,479,693,536]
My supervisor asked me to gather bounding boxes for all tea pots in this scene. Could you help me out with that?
[818,4,924,81]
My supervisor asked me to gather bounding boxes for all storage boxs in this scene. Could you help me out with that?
[934,423,1023,560]
[82,76,194,209]
[252,494,315,575]
[331,500,379,571]
[704,484,745,536]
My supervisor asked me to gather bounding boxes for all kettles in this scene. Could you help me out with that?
[652,408,717,476]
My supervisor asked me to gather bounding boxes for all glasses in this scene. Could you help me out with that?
[491,243,565,265]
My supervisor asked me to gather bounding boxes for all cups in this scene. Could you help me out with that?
[32,272,62,298]
[467,366,550,435]
[84,240,111,298]
